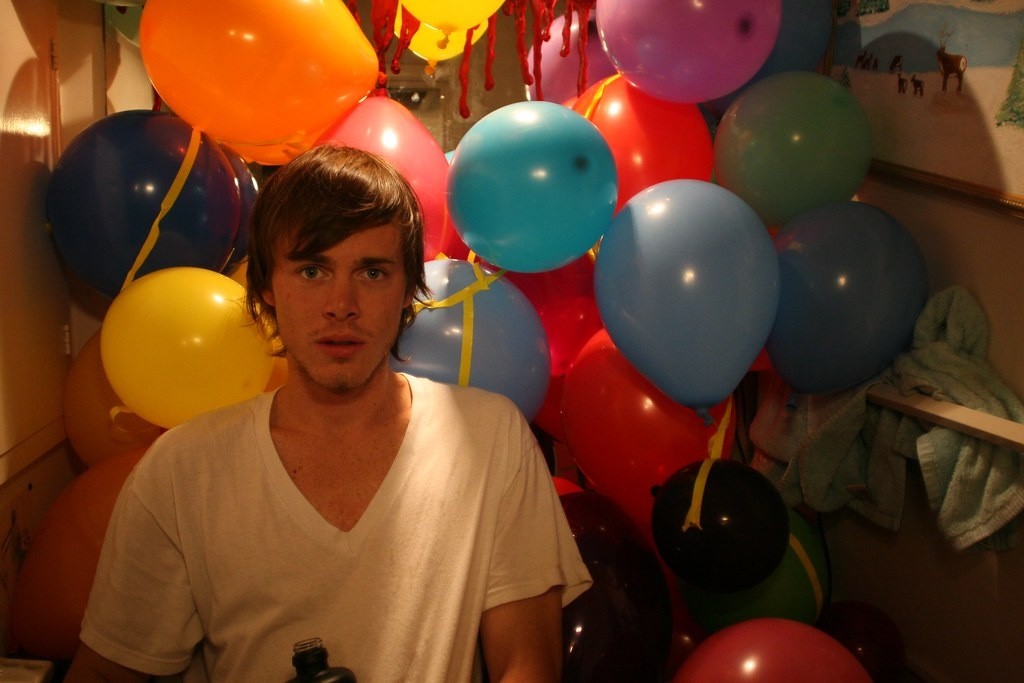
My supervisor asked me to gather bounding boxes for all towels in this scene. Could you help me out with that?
[745,283,1024,553]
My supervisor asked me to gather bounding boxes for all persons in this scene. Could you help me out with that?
[62,145,593,682]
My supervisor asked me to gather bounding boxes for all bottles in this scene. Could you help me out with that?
[285,638,356,683]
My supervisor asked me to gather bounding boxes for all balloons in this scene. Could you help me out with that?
[34,0,929,683]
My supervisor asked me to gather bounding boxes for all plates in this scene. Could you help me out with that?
[0,657,52,683]
